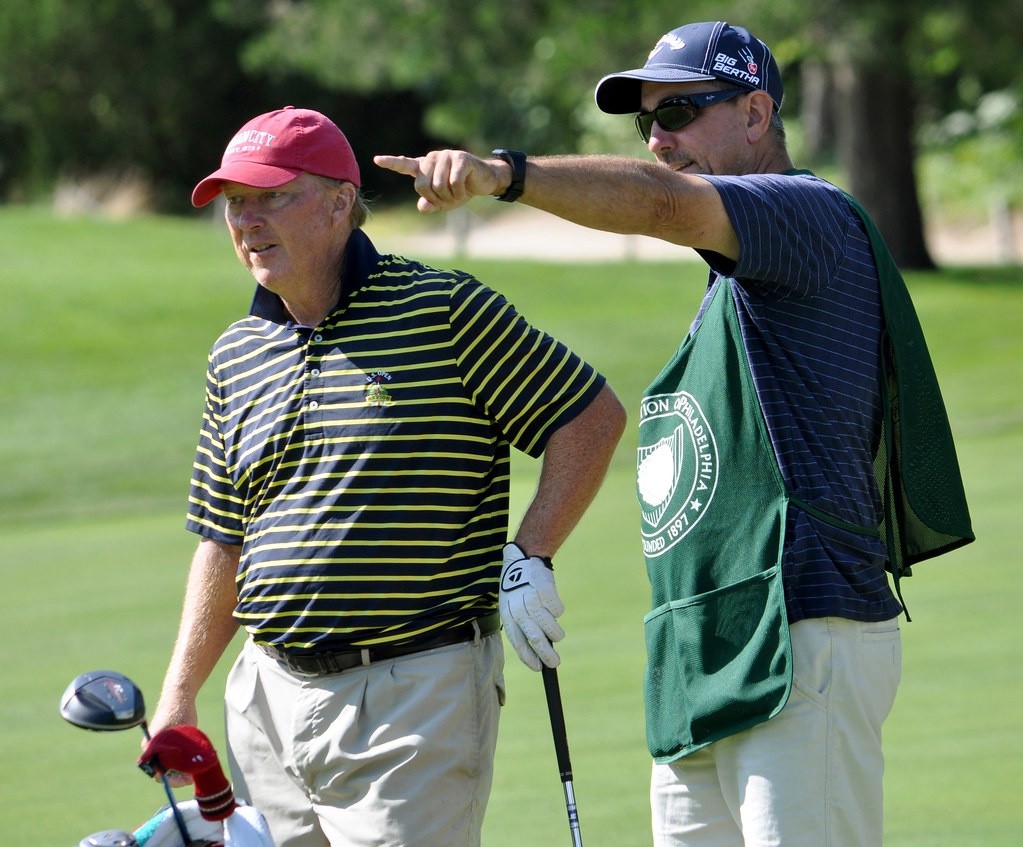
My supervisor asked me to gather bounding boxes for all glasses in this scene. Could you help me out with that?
[635,88,752,144]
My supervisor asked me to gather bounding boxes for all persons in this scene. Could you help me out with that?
[374,21,976,847]
[140,106,628,847]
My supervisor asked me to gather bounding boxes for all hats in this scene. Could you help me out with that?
[594,21,785,115]
[191,105,359,209]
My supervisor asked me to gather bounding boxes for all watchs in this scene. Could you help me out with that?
[492,149,526,203]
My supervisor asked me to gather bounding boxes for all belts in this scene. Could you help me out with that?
[257,612,501,676]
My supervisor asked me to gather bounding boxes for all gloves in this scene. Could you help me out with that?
[501,541,566,670]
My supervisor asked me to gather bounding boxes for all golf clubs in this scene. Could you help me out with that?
[540,634,582,847]
[59,670,193,847]
[80,830,139,847]
